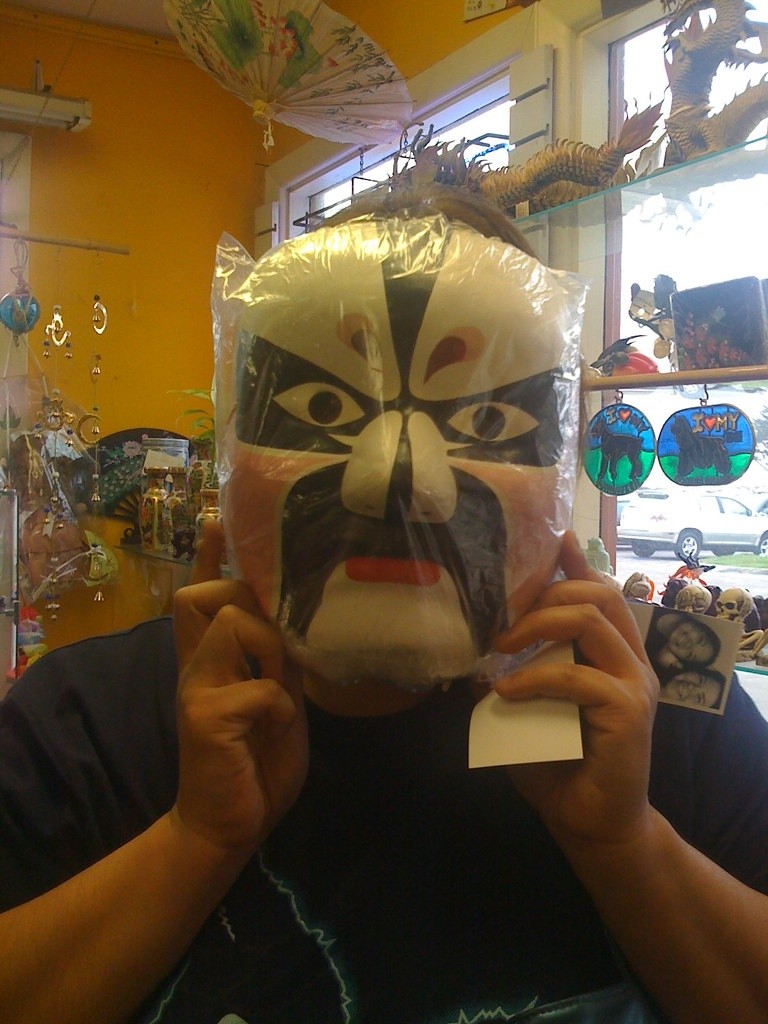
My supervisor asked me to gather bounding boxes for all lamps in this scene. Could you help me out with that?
[0,87,93,133]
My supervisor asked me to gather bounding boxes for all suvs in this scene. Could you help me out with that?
[617,488,768,561]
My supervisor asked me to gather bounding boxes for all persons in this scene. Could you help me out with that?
[644,606,726,710]
[0,183,768,1023]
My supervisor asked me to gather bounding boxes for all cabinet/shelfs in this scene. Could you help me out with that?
[512,127,768,676]
